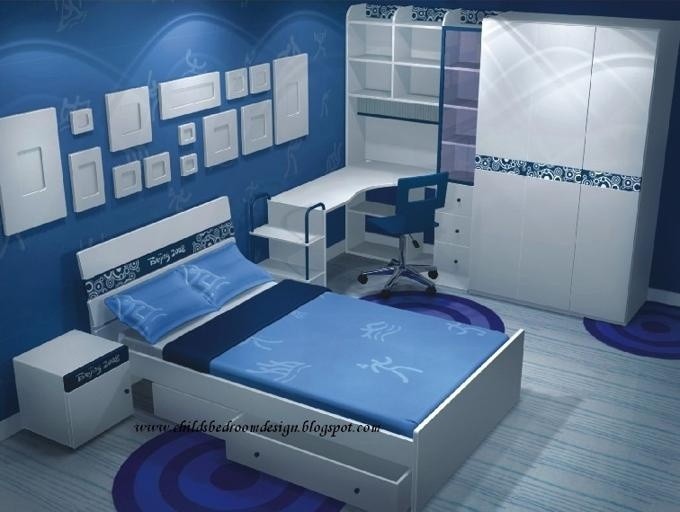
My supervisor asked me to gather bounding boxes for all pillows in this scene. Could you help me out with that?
[104,241,276,345]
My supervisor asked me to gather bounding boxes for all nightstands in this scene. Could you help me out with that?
[13,328,135,449]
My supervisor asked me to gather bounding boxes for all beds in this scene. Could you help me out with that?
[76,196,525,512]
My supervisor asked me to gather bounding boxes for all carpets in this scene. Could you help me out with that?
[356,290,505,334]
[583,300,680,359]
[111,424,346,512]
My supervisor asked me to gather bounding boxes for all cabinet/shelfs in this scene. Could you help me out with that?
[248,4,680,326]
